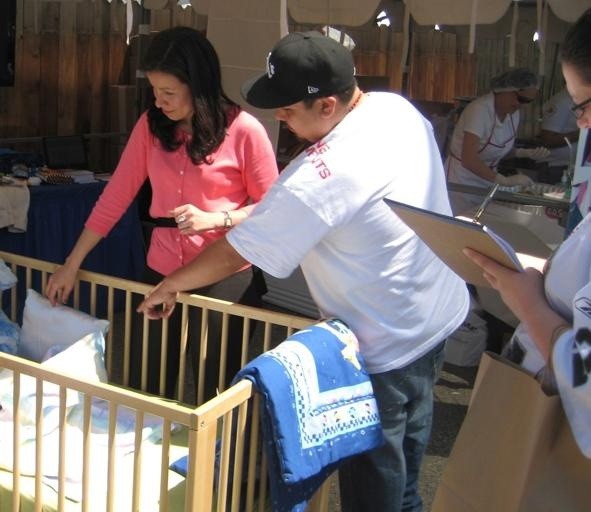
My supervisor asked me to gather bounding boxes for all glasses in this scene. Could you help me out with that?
[569,97,591,119]
[512,90,534,105]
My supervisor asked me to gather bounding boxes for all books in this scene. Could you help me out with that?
[382,197,528,286]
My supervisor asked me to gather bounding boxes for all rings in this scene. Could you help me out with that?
[179,215,185,221]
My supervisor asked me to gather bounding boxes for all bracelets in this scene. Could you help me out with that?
[222,211,232,228]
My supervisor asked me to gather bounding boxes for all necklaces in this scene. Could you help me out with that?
[348,90,363,112]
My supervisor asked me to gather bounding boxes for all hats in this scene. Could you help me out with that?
[239,29,358,110]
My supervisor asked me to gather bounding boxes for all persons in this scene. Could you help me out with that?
[136,30,470,512]
[45,27,279,405]
[443,70,580,217]
[463,7,591,460]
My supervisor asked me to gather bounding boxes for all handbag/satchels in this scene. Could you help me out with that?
[419,321,590,511]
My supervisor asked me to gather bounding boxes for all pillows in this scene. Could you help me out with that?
[0,263,111,443]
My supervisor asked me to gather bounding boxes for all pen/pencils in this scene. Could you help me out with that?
[472,183,499,224]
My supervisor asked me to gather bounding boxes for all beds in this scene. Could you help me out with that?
[0,249,345,512]
